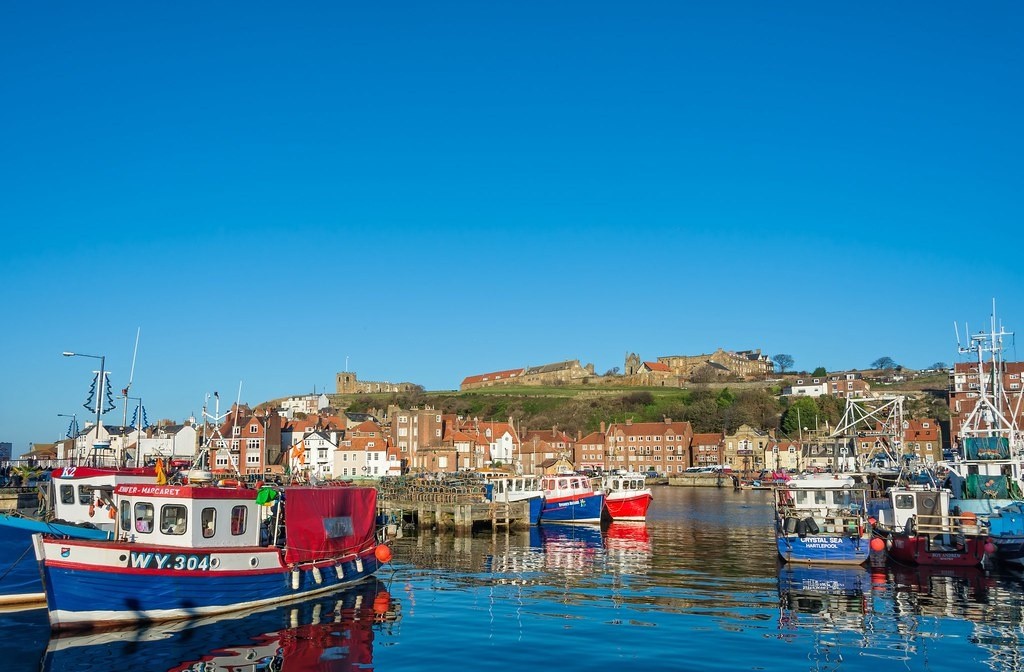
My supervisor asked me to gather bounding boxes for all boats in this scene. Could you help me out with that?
[521,433,608,526]
[471,460,545,527]
[776,558,872,615]
[0,579,380,672]
[771,297,1024,566]
[594,472,653,521]
[0,372,397,633]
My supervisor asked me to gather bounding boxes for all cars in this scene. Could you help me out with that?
[579,470,658,478]
[685,467,719,474]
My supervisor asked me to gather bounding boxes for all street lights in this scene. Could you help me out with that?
[63,352,104,466]
[116,396,142,468]
[58,413,75,469]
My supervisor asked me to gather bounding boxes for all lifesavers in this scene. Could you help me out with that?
[216,478,246,488]
[859,516,865,537]
